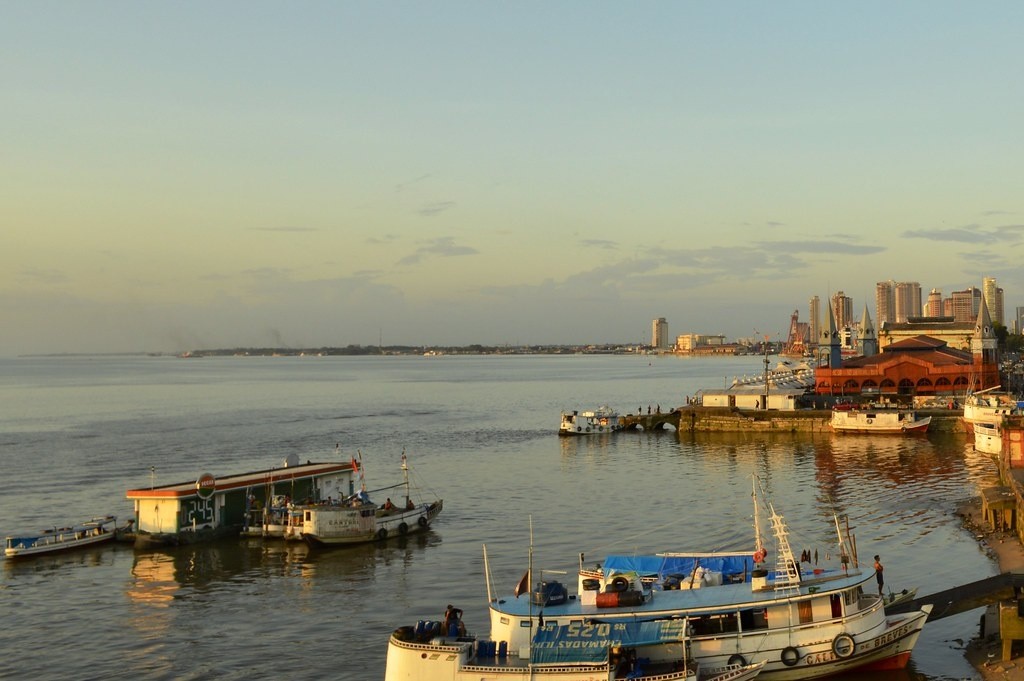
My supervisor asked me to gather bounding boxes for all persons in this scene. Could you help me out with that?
[874,555,885,595]
[685,395,699,406]
[638,404,674,416]
[812,397,857,409]
[407,500,414,509]
[755,399,760,411]
[384,498,395,510]
[444,605,465,637]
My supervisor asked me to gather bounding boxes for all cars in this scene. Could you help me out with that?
[922,400,948,408]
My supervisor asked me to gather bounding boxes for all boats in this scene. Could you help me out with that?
[384,470,934,681]
[828,410,933,434]
[2,515,135,562]
[558,405,625,434]
[239,451,444,549]
[118,449,367,547]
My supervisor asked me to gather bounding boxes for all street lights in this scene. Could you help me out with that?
[765,349,776,407]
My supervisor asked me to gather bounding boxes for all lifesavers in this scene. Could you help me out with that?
[728,654,746,669]
[867,419,872,424]
[781,646,800,666]
[599,426,603,431]
[751,570,768,577]
[419,517,427,526]
[399,522,408,534]
[901,427,907,433]
[586,427,590,431]
[378,528,387,539]
[578,427,581,431]
[612,577,628,592]
[832,632,856,659]
[753,552,763,562]
[760,547,767,557]
[611,426,615,429]
[617,425,621,429]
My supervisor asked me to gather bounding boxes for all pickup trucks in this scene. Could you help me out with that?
[832,400,859,411]
